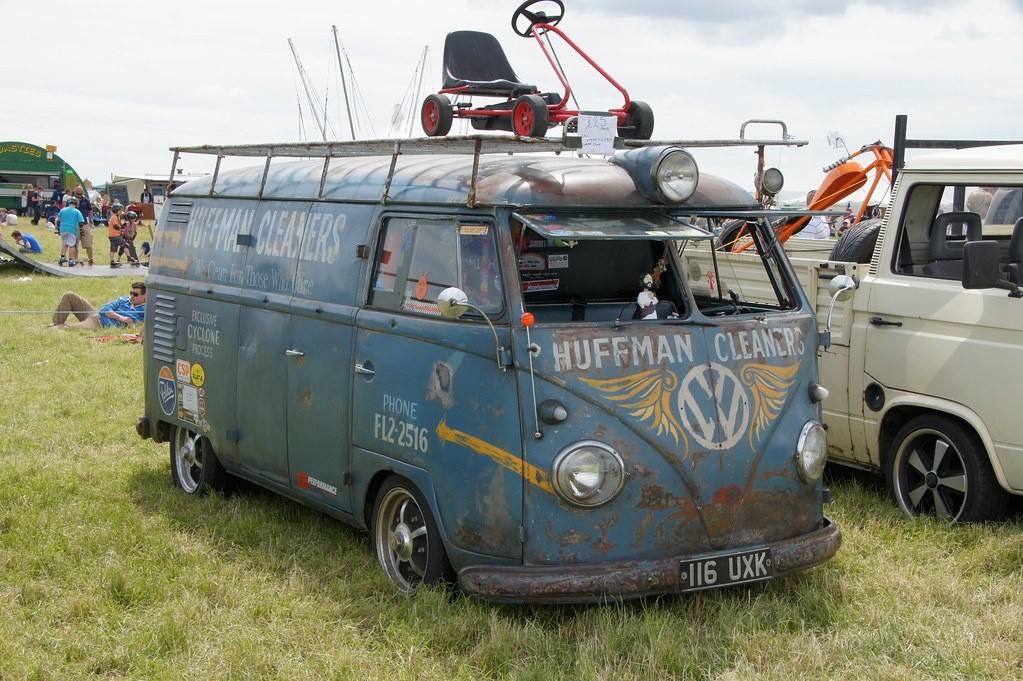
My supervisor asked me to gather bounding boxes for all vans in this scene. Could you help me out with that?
[137,131,855,613]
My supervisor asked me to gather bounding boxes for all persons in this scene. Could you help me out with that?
[962,186,997,227]
[0,180,158,267]
[798,190,830,238]
[49,282,145,330]
[829,204,881,237]
[637,272,658,320]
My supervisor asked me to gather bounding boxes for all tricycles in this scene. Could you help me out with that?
[418,0,657,145]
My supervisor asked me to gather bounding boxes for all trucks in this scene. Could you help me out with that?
[666,143,1022,528]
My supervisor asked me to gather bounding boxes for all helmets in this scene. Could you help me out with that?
[68,197,79,204]
[126,211,138,218]
[112,204,122,213]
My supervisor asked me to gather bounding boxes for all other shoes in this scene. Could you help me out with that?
[127,256,135,262]
[110,260,123,268]
[69,259,76,266]
[88,259,94,266]
[60,257,68,266]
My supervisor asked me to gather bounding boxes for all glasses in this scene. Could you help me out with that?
[130,291,142,297]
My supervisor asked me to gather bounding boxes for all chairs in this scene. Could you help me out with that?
[927,212,984,282]
[440,28,538,92]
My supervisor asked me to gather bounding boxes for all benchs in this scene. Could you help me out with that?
[526,300,681,325]
